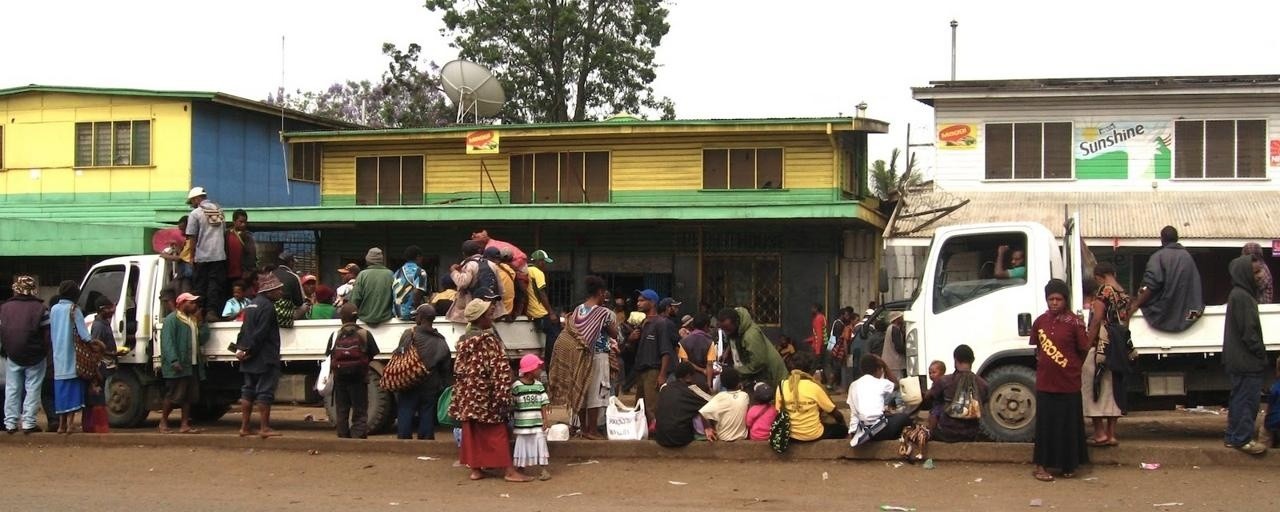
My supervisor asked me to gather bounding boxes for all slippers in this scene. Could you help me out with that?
[156,424,174,435]
[1032,468,1054,481]
[1109,440,1120,447]
[1085,437,1109,447]
[178,427,199,434]
[1062,469,1076,478]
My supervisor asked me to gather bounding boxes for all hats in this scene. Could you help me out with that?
[694,312,713,327]
[681,314,694,327]
[659,297,682,308]
[635,288,659,304]
[257,272,284,294]
[93,296,113,311]
[888,310,903,324]
[474,287,502,302]
[12,275,40,296]
[530,249,554,264]
[365,247,384,264]
[299,273,317,287]
[410,303,436,320]
[56,279,81,298]
[464,298,491,322]
[337,263,360,275]
[337,303,358,316]
[516,353,545,373]
[185,186,207,205]
[316,285,335,303]
[483,246,501,258]
[754,381,773,403]
[176,293,201,303]
[865,308,875,316]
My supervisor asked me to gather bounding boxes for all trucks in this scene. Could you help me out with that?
[877,205,1279,442]
[73,251,550,436]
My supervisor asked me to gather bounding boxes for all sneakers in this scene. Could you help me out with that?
[1223,441,1233,448]
[22,425,43,433]
[1237,440,1266,455]
[6,428,18,434]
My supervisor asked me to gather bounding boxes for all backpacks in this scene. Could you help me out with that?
[461,255,498,296]
[331,325,365,378]
[944,372,982,420]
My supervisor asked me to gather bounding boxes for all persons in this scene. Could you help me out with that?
[994,245,1026,279]
[1080,260,1132,447]
[1030,280,1090,482]
[1129,225,1206,332]
[1222,253,1269,454]
[1241,243,1274,304]
[1262,357,1280,448]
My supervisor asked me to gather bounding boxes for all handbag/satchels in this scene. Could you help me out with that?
[202,207,225,227]
[768,410,791,455]
[812,325,829,346]
[826,335,836,351]
[603,395,651,441]
[74,333,106,380]
[380,345,433,392]
[832,336,845,362]
[316,353,334,397]
